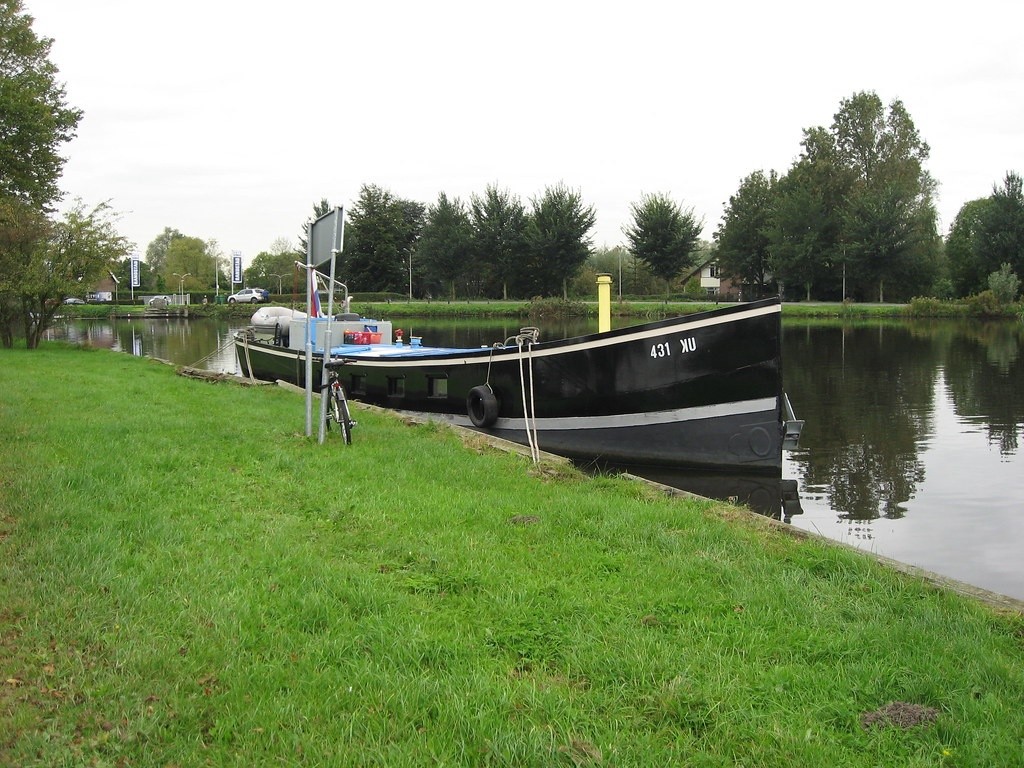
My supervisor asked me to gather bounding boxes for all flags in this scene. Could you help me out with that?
[311,268,327,319]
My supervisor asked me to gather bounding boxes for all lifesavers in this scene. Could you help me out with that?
[279,323,289,348]
[465,386,497,429]
[335,313,362,321]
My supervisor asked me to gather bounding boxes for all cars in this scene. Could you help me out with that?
[148,295,172,307]
[227,287,264,304]
[63,298,87,305]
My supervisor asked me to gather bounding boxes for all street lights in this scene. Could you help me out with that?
[173,273,191,305]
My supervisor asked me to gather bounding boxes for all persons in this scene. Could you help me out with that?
[262,290,269,300]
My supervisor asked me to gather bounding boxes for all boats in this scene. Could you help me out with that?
[233,262,806,466]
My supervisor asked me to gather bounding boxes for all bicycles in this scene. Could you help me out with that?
[324,358,356,445]
